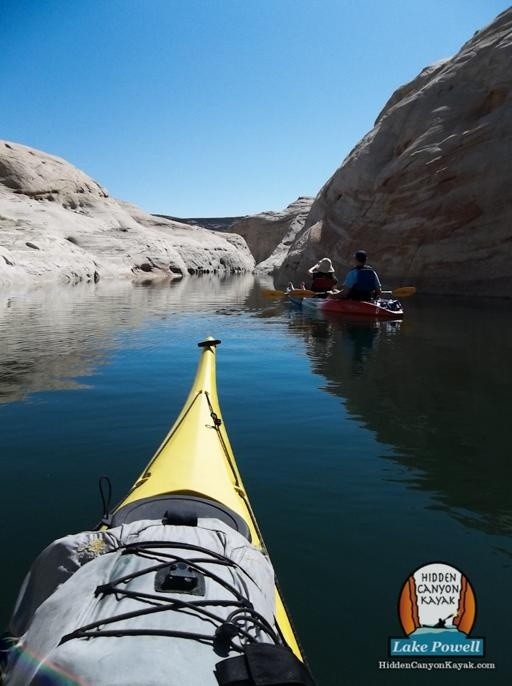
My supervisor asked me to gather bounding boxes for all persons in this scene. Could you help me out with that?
[306,319,335,342]
[342,323,382,376]
[288,257,338,294]
[326,250,382,303]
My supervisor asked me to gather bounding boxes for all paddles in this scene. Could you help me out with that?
[262,290,290,301]
[290,286,416,298]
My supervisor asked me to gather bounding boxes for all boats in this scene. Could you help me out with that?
[285,285,405,318]
[0,336,308,686]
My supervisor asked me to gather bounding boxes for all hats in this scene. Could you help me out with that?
[316,257,335,273]
